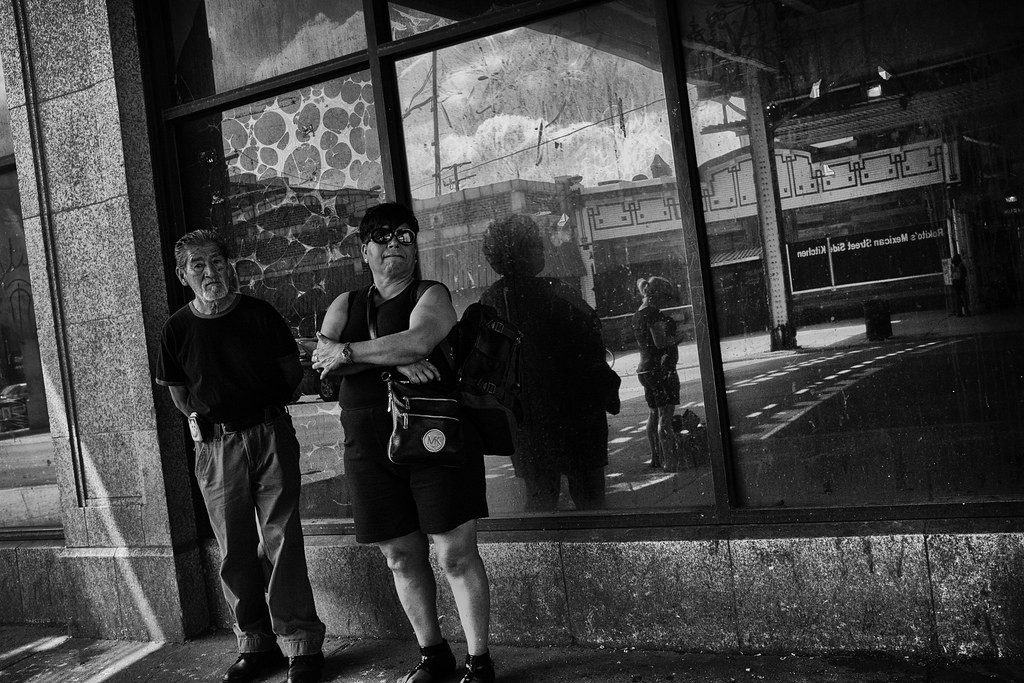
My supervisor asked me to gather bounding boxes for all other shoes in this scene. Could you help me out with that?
[460,663,496,683]
[397,650,456,683]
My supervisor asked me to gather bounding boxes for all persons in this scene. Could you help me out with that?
[311,201,496,683]
[475,217,689,513]
[154,230,327,683]
[951,255,971,317]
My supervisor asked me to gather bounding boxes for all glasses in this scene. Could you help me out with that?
[366,228,417,245]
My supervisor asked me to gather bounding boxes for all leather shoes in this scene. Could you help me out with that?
[287,650,325,683]
[222,643,283,683]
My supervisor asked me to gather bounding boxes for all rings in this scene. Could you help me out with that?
[316,356,319,363]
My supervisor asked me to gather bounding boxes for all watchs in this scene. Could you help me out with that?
[342,343,354,365]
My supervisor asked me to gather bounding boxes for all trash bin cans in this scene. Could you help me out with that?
[859,293,894,340]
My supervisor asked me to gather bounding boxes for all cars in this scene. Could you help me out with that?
[0,383,30,427]
[286,337,341,405]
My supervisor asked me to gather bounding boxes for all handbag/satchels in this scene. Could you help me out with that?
[669,410,709,468]
[382,371,461,466]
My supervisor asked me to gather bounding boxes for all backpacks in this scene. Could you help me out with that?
[412,280,528,456]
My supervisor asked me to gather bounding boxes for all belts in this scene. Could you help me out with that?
[212,405,286,436]
